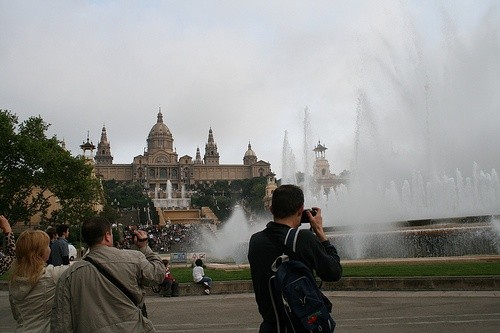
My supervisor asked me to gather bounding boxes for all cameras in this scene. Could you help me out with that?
[301,209,316,223]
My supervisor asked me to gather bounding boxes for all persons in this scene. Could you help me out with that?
[161,260,177,297]
[46,225,70,266]
[247,184,343,333]
[0,215,16,277]
[55,215,166,333]
[8,230,70,333]
[121,223,199,252]
[192,259,212,295]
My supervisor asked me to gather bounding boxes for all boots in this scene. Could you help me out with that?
[171,283,181,297]
[163,279,172,297]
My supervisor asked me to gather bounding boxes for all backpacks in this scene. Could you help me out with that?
[268,228,336,333]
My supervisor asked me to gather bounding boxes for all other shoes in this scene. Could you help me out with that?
[205,290,210,295]
[204,282,209,286]
